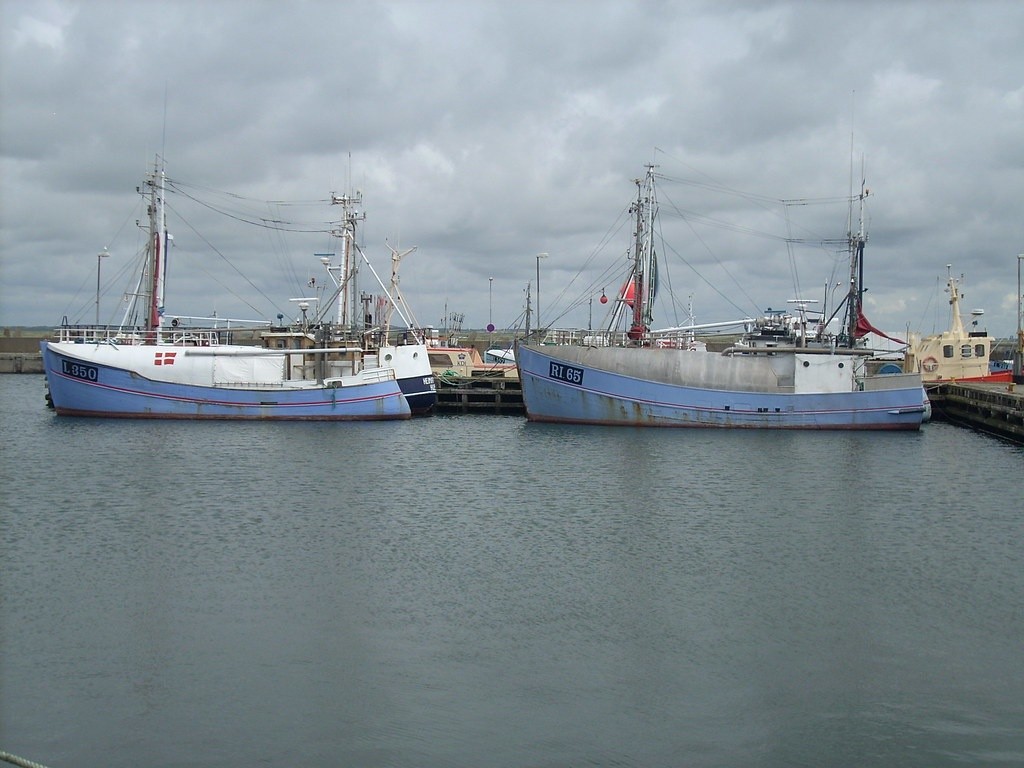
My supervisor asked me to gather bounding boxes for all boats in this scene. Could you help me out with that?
[505,331,933,437]
[904,262,1014,383]
[36,77,934,378]
[40,329,442,424]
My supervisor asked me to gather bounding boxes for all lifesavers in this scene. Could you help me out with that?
[923,356,939,372]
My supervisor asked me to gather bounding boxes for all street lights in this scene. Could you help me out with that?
[535,252,549,328]
[95,253,111,336]
